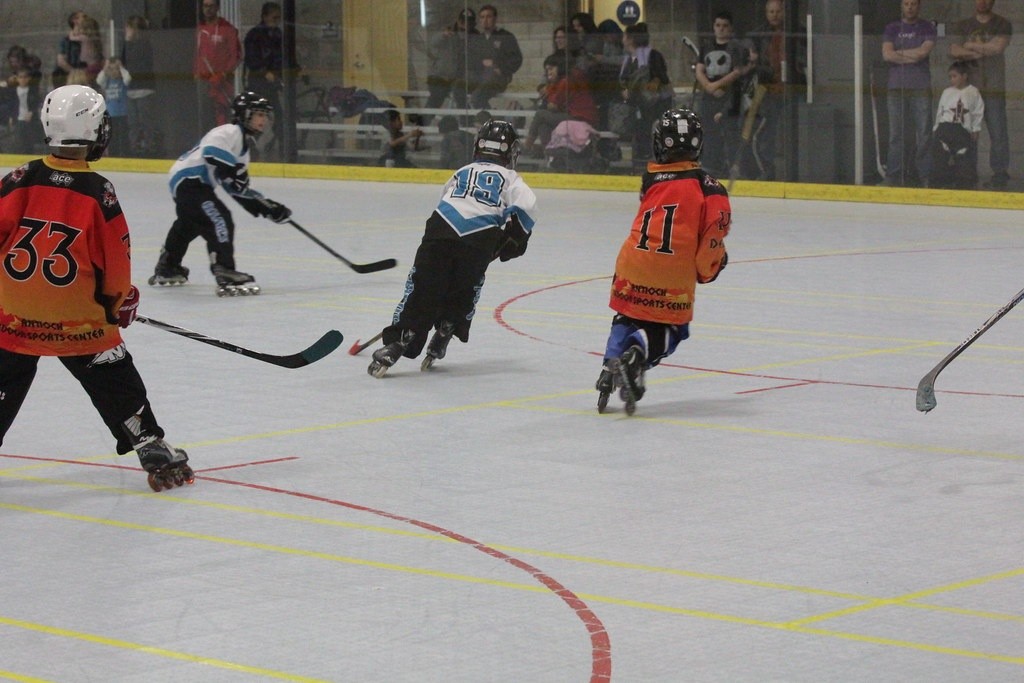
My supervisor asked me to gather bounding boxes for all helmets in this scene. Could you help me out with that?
[230,93,276,139]
[651,109,706,164]
[474,118,521,169]
[41,84,112,161]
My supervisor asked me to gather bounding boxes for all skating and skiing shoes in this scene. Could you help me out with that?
[209,264,260,298]
[147,250,190,286]
[122,414,194,492]
[607,347,647,416]
[595,357,616,414]
[420,318,457,370]
[367,327,417,378]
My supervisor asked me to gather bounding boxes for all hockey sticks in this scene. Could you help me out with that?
[132,313,345,371]
[915,287,1024,412]
[348,236,519,355]
[251,184,397,275]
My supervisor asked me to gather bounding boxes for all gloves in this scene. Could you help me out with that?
[229,162,249,195]
[499,214,532,263]
[114,285,140,328]
[259,200,293,224]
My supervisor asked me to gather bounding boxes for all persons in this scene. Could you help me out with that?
[149,90,292,297]
[596,109,732,415]
[326,86,425,168]
[0,84,194,492]
[928,60,985,191]
[950,1,1011,191]
[194,0,242,139]
[518,12,674,174]
[406,5,523,126]
[695,0,807,177]
[244,2,283,163]
[0,45,42,152]
[55,10,157,158]
[438,111,492,169]
[875,0,938,187]
[368,118,538,378]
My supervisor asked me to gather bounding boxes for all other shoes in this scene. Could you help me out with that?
[990,169,1009,187]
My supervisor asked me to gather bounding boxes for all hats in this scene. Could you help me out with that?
[460,8,476,21]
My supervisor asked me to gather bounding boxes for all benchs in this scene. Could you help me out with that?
[296,87,700,170]
